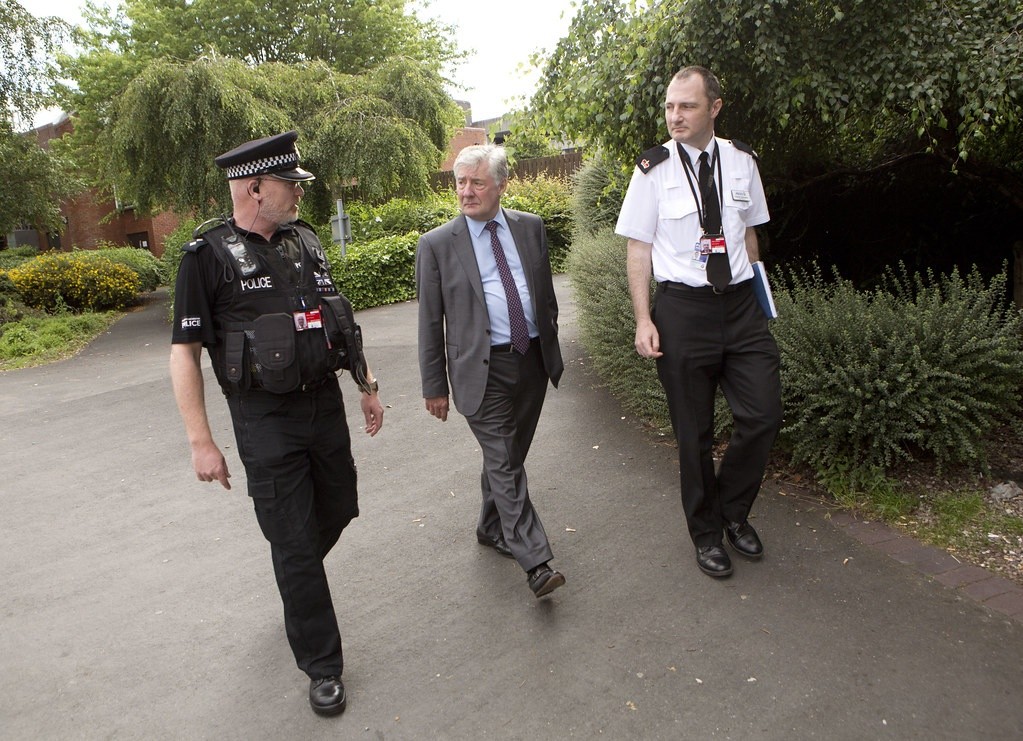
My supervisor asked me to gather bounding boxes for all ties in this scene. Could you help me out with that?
[698,152,732,288]
[485,220,530,356]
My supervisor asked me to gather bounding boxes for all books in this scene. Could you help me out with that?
[750,261,778,321]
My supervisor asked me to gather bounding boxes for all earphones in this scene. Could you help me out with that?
[253,186,260,195]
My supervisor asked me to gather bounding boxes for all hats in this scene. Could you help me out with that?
[214,131,316,183]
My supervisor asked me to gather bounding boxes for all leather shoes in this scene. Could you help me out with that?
[526,562,564,597]
[721,516,764,557]
[308,674,343,715]
[695,541,734,576]
[477,528,515,558]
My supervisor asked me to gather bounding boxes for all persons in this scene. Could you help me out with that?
[296,315,306,330]
[613,66,782,577]
[702,240,711,253]
[417,145,566,598]
[171,127,384,717]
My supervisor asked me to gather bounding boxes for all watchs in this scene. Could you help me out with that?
[358,378,380,393]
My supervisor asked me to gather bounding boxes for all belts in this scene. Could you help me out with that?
[490,336,541,353]
[662,279,752,295]
